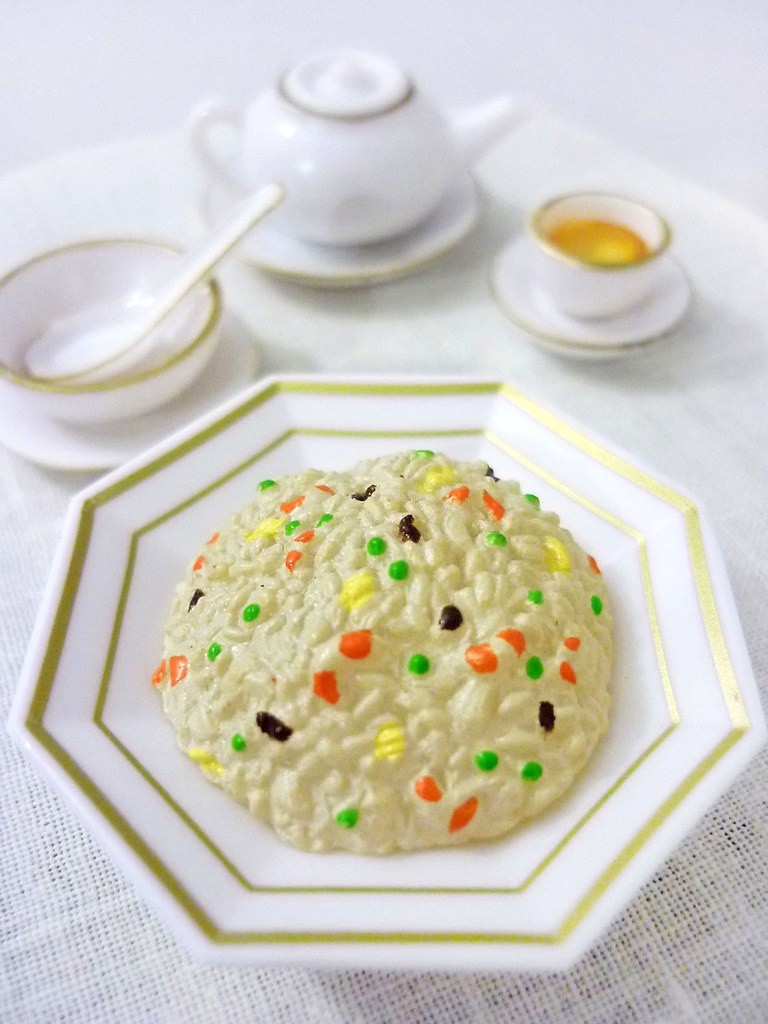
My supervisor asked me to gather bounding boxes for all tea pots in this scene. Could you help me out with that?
[186,50,524,248]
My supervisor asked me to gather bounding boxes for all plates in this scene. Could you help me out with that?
[487,239,694,357]
[0,309,261,468]
[6,376,764,977]
[201,175,482,287]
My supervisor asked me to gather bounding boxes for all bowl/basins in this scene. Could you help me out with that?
[0,240,226,424]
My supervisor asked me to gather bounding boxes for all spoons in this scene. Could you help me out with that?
[26,182,285,380]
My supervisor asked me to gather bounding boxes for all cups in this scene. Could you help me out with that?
[528,190,671,321]
